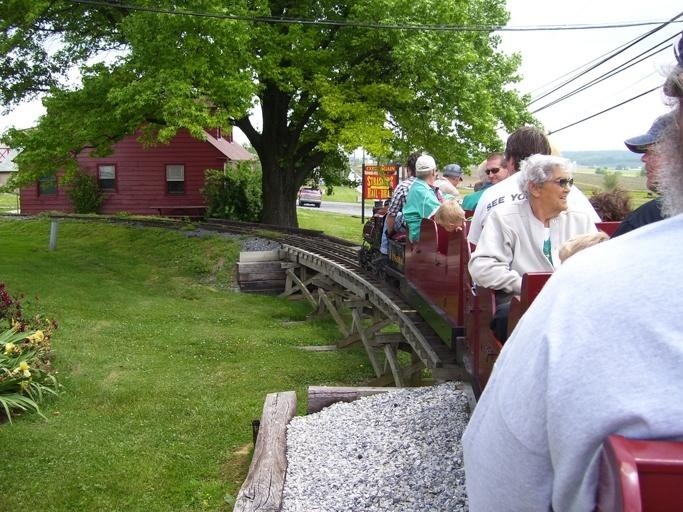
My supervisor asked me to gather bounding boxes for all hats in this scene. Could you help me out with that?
[415,155,436,171]
[623,114,670,154]
[442,164,464,181]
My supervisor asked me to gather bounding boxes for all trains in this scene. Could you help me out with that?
[352,180,683,512]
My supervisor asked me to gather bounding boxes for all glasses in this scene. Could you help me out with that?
[544,178,573,188]
[484,168,499,175]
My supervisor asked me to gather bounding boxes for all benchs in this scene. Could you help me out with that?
[149,205,210,220]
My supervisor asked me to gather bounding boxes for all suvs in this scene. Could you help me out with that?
[298,186,321,208]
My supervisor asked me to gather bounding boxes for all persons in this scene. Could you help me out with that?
[461,40,683,511]
[611,109,678,240]
[376,127,610,346]
